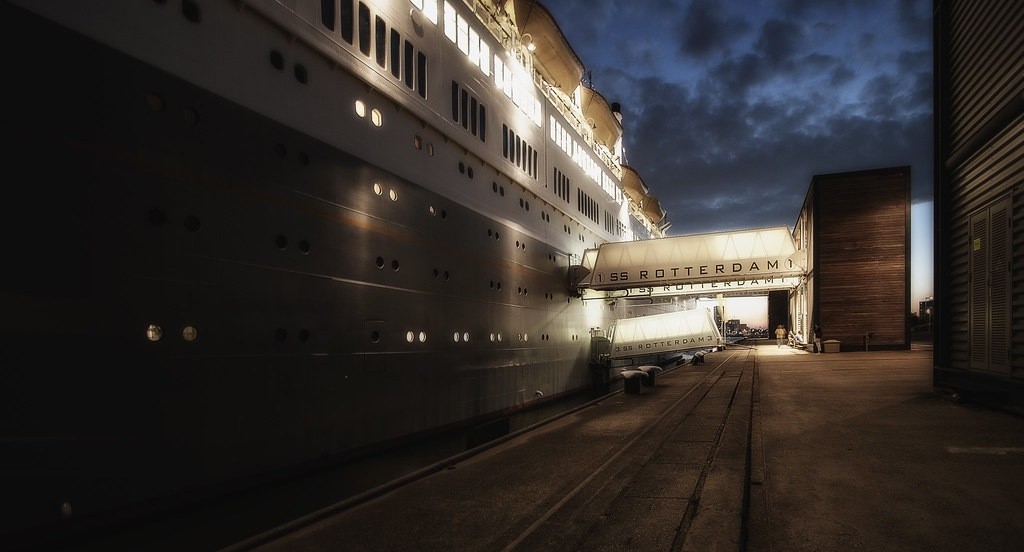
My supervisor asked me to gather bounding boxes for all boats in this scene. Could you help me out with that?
[621,163,650,205]
[513,0,586,98]
[579,81,624,151]
[642,193,667,225]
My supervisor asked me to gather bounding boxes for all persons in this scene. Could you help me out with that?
[775,324,804,350]
[814,323,822,356]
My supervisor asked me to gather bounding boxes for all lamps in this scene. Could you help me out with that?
[584,117,596,130]
[518,33,536,59]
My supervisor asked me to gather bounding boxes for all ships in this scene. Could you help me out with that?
[0,0,668,552]
